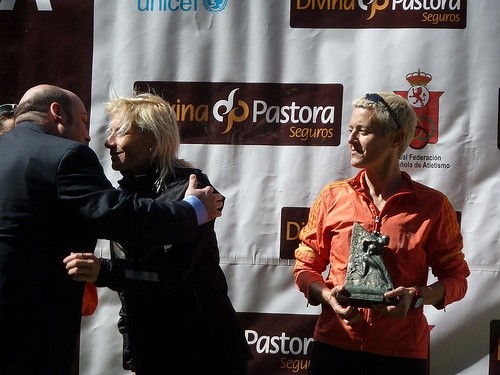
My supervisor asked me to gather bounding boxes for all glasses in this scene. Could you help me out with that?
[0,104,18,114]
[365,93,402,129]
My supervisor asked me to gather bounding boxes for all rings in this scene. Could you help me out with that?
[80,253,85,259]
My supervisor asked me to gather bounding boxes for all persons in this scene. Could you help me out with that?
[0,84,223,375]
[63,92,254,375]
[350,234,393,290]
[293,93,470,375]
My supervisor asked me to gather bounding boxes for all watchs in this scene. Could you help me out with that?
[411,286,425,309]
